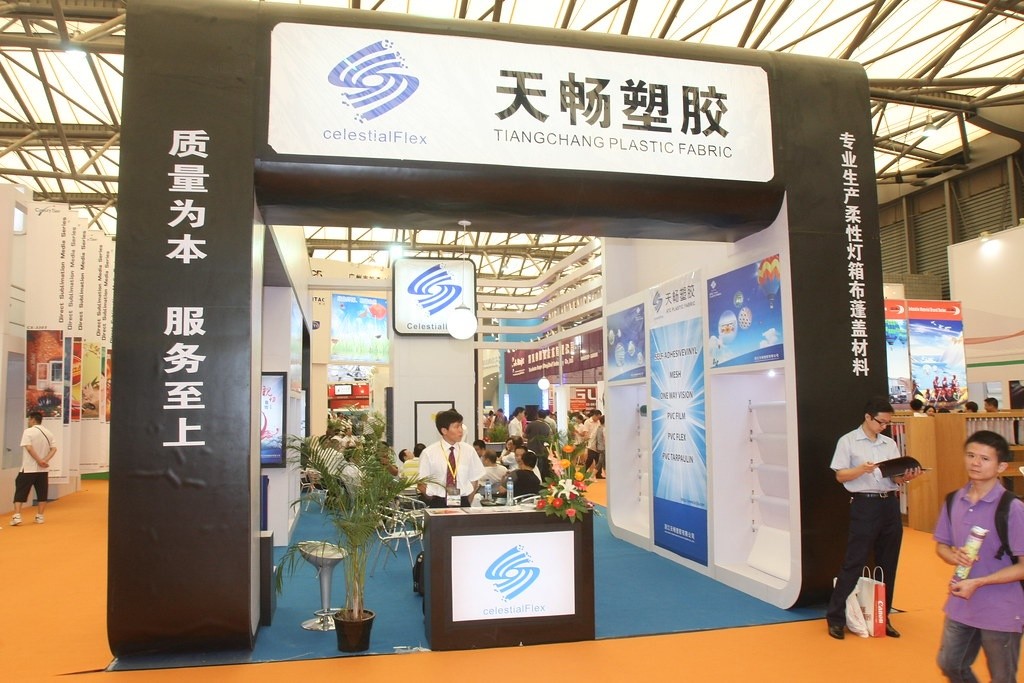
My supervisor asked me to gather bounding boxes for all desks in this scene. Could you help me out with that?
[423,505,595,652]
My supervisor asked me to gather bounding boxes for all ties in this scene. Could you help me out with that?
[445,447,457,498]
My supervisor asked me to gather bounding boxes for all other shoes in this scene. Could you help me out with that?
[595,473,606,479]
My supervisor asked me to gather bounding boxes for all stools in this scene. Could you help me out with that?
[297,541,348,631]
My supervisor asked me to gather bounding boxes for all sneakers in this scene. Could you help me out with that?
[10,517,22,525]
[34,516,44,524]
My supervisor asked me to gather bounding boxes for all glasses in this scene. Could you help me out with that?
[870,415,892,427]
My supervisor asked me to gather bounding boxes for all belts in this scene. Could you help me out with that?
[853,492,896,498]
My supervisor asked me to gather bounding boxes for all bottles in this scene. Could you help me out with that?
[507,476,514,506]
[485,478,493,499]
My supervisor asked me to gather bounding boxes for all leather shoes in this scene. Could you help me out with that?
[883,620,901,636]
[827,617,845,639]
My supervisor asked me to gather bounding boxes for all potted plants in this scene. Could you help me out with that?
[274,410,448,652]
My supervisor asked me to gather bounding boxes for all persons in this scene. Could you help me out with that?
[473,406,606,501]
[984,398,999,412]
[306,411,426,523]
[933,430,1024,683]
[826,398,924,640]
[933,375,959,397]
[417,408,486,625]
[10,412,57,526]
[965,401,978,412]
[910,399,950,413]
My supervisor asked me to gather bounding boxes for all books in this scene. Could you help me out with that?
[875,455,933,478]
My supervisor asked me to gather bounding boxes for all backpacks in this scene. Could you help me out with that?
[413,551,425,591]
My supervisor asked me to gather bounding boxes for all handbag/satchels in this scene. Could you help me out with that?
[833,566,887,637]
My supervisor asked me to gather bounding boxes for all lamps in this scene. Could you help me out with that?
[447,218,478,339]
[980,232,988,242]
[923,114,938,134]
[538,347,551,391]
[66,29,87,57]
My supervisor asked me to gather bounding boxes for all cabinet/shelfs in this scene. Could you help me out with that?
[889,410,1024,534]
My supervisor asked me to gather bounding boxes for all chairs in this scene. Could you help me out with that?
[300,468,428,577]
[512,493,540,505]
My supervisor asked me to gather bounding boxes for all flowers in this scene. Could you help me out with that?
[529,422,603,524]
